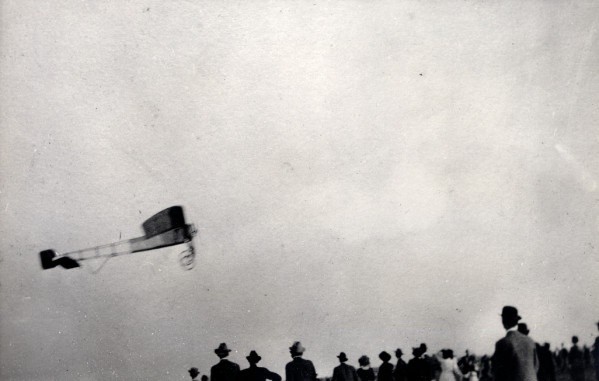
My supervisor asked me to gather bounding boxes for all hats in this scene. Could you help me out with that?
[187,367,200,374]
[336,352,348,360]
[379,350,391,360]
[214,343,231,353]
[420,344,427,349]
[518,324,529,333]
[498,307,521,319]
[393,348,404,354]
[288,342,306,352]
[358,355,370,364]
[246,350,261,359]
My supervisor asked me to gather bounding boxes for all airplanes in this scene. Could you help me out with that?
[39,206,198,273]
